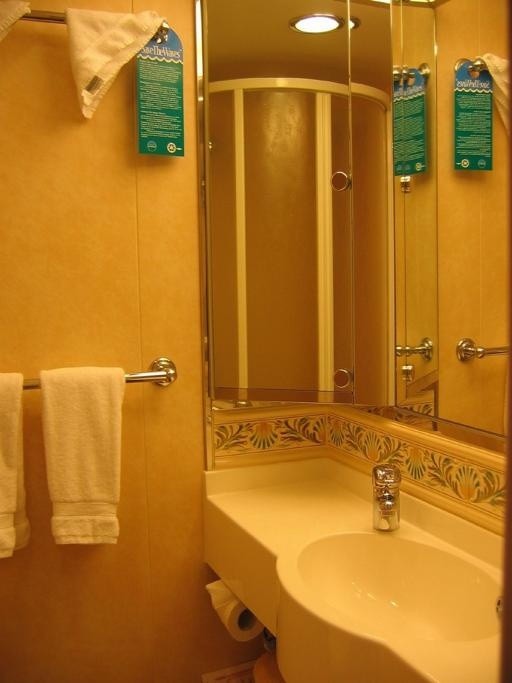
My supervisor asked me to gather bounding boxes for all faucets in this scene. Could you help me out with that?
[371,465,402,531]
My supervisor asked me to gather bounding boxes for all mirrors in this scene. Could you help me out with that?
[196,2,512,454]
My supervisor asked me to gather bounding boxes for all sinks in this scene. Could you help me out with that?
[293,525,505,649]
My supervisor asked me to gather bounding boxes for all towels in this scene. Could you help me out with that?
[66,4,174,117]
[1,366,134,557]
